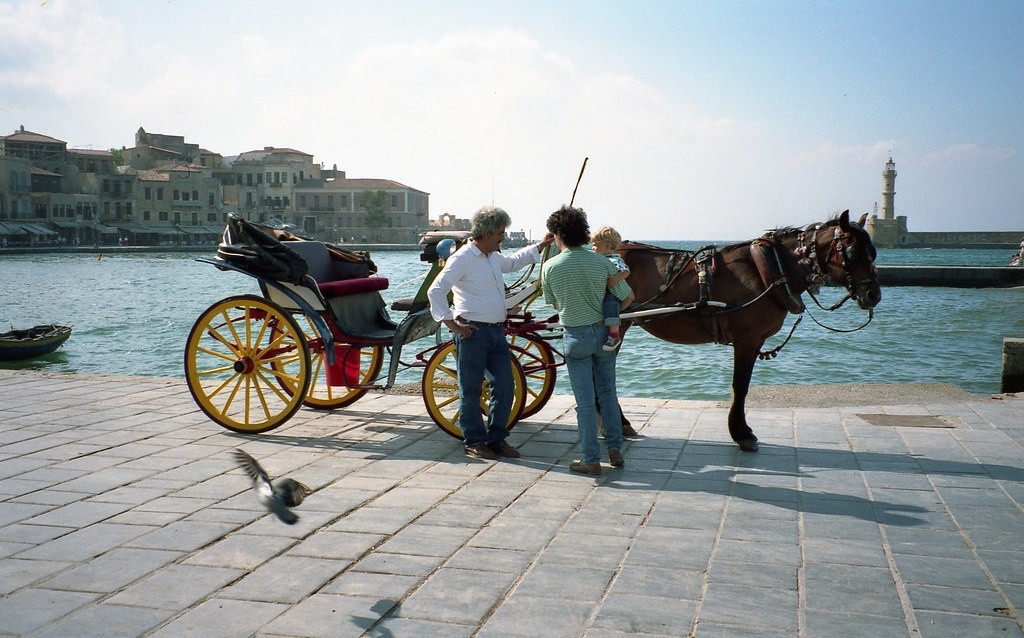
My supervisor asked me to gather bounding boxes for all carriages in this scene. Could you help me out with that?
[183,208,883,453]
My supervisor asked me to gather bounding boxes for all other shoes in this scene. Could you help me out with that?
[609,450,624,466]
[465,444,495,459]
[570,460,601,475]
[487,440,519,457]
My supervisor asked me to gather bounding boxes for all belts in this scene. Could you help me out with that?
[457,316,505,327]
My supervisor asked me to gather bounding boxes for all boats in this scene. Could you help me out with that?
[0,323,73,364]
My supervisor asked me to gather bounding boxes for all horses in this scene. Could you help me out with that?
[594,209,884,453]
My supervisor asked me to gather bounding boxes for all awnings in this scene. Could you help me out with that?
[84,222,225,234]
[0,222,57,235]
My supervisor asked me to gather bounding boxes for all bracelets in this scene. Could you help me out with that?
[537,243,542,251]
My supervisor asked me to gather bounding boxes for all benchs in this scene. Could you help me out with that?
[391,257,437,311]
[278,238,390,297]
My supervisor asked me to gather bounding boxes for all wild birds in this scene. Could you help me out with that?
[231,450,307,526]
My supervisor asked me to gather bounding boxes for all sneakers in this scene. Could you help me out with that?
[602,335,621,352]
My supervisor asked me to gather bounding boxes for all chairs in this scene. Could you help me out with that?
[500,277,538,309]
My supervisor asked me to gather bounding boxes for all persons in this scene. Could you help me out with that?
[591,225,630,351]
[427,207,554,458]
[540,207,635,473]
[1011,241,1024,266]
